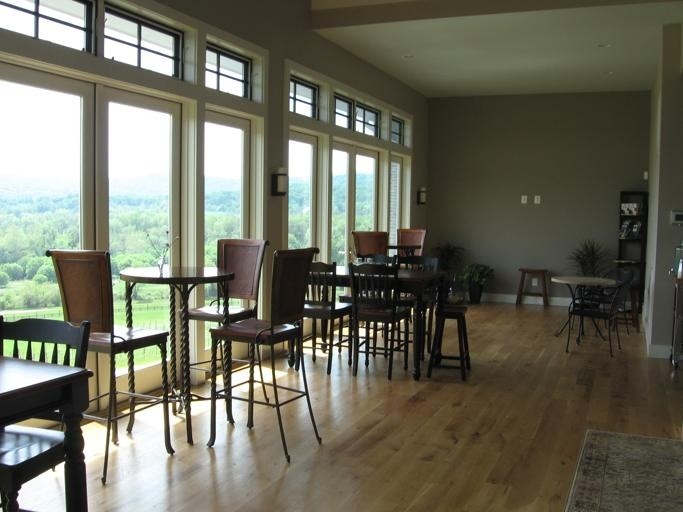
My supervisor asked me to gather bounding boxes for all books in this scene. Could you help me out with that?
[619,219,641,237]
[620,203,638,214]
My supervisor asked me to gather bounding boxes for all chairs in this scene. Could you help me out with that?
[178,239,269,422]
[207,247,321,462]
[0,315,91,512]
[295,229,442,381]
[46,249,174,484]
[565,268,640,357]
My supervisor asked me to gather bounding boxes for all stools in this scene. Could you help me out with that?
[515,268,548,308]
[427,306,471,382]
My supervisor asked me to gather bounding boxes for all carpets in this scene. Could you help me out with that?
[564,429,683,512]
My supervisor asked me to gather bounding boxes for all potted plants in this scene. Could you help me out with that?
[466,264,494,304]
[434,242,465,304]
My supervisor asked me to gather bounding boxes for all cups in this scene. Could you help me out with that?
[366,258,372,263]
[354,258,362,263]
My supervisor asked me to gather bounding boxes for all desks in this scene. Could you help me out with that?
[120,266,235,454]
[551,276,617,342]
[0,355,87,512]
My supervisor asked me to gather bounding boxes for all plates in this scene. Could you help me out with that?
[353,263,363,266]
[364,262,375,265]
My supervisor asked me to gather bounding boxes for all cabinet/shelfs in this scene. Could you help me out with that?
[617,191,648,313]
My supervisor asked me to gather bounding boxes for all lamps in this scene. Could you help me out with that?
[271,168,288,196]
[416,187,426,204]
